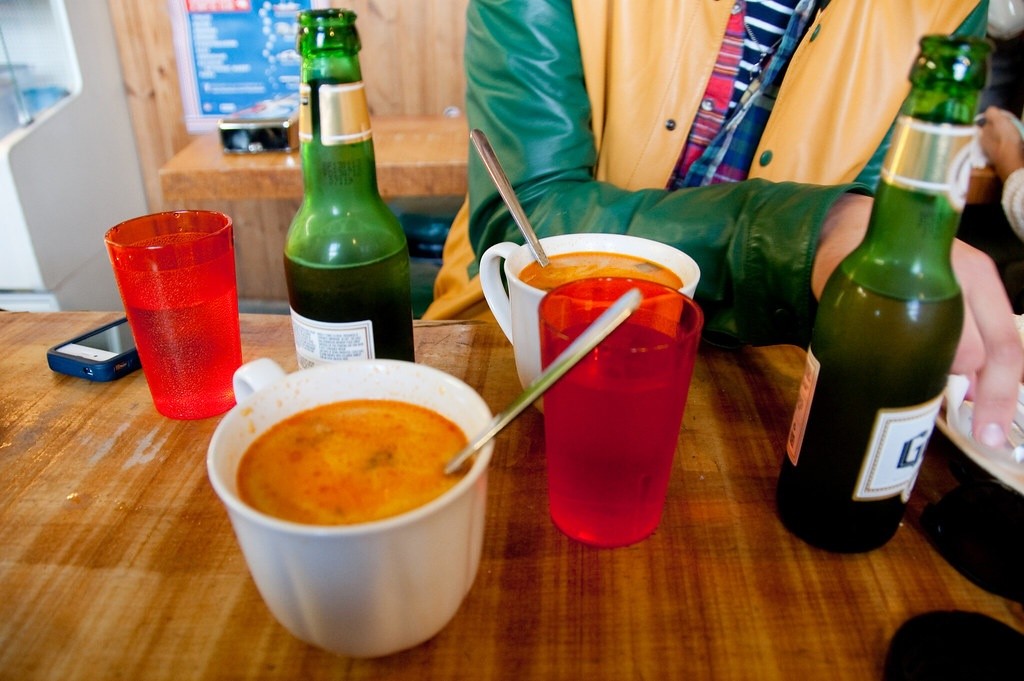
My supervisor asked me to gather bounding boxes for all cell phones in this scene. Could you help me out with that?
[47,317,142,383]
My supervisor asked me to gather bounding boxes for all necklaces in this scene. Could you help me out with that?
[741,19,783,82]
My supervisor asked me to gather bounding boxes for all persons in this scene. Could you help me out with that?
[419,0,1024,453]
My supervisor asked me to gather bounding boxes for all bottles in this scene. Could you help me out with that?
[776,36,993,554]
[283,6,415,372]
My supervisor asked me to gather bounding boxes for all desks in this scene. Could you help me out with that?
[0,311,1023,681]
[158,112,468,243]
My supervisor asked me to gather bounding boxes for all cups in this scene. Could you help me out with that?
[480,231,703,417]
[533,277,703,547]
[207,351,498,657]
[104,210,245,421]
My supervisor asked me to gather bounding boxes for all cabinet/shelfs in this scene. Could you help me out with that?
[0,0,149,290]
